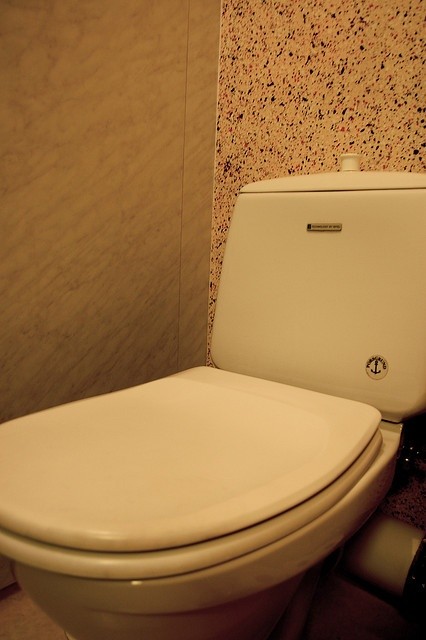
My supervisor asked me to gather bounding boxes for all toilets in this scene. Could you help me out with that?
[0,172,426,640]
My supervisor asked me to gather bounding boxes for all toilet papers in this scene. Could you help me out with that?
[342,510,426,598]
